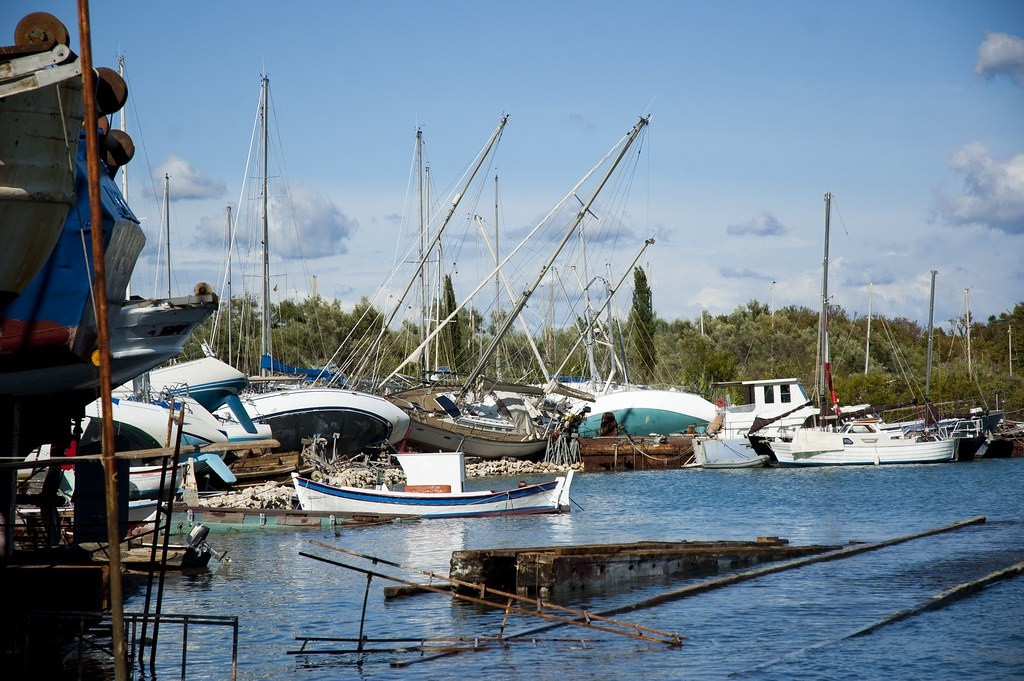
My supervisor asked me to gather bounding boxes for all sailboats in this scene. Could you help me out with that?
[33,52,1024,471]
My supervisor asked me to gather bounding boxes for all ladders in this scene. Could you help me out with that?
[285,537,684,657]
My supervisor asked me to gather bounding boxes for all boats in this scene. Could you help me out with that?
[0,12,222,534]
[290,444,573,520]
